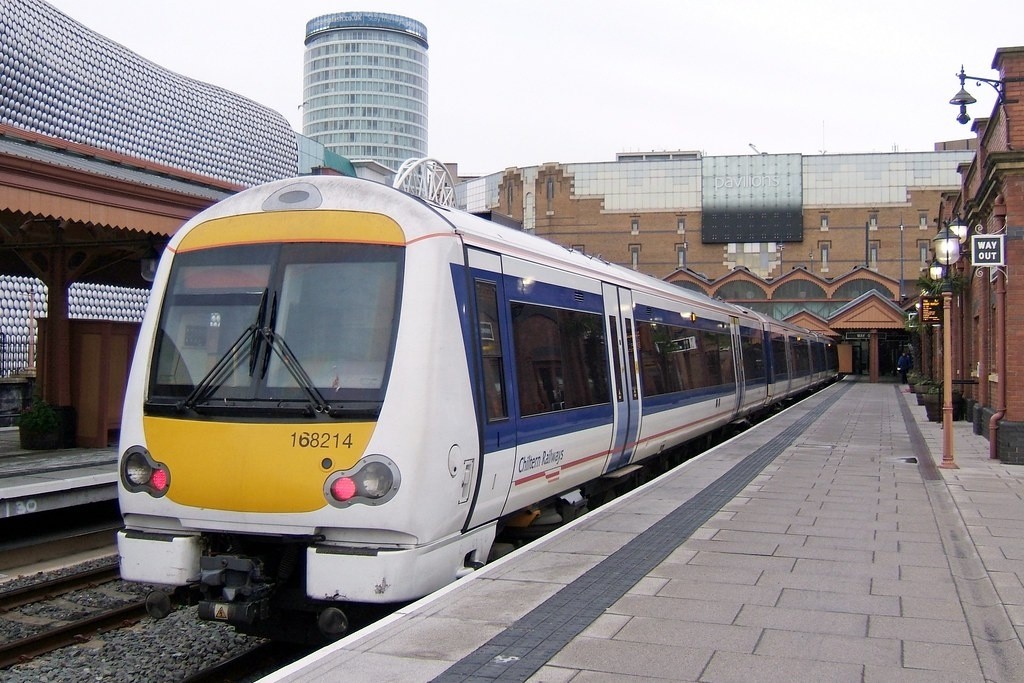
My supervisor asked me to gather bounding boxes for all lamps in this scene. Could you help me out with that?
[140,242,161,281]
[949,212,972,259]
[949,64,1005,124]
[18,217,34,236]
[932,220,973,265]
[58,220,71,234]
[929,259,945,280]
[915,300,920,311]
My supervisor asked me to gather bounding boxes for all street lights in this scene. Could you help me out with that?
[930,220,960,470]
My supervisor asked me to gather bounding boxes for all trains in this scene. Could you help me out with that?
[111,172,841,647]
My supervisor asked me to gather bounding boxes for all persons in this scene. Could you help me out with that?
[898,351,912,384]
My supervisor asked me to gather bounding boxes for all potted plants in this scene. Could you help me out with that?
[922,386,960,422]
[914,378,941,406]
[907,372,930,393]
[19,398,62,450]
